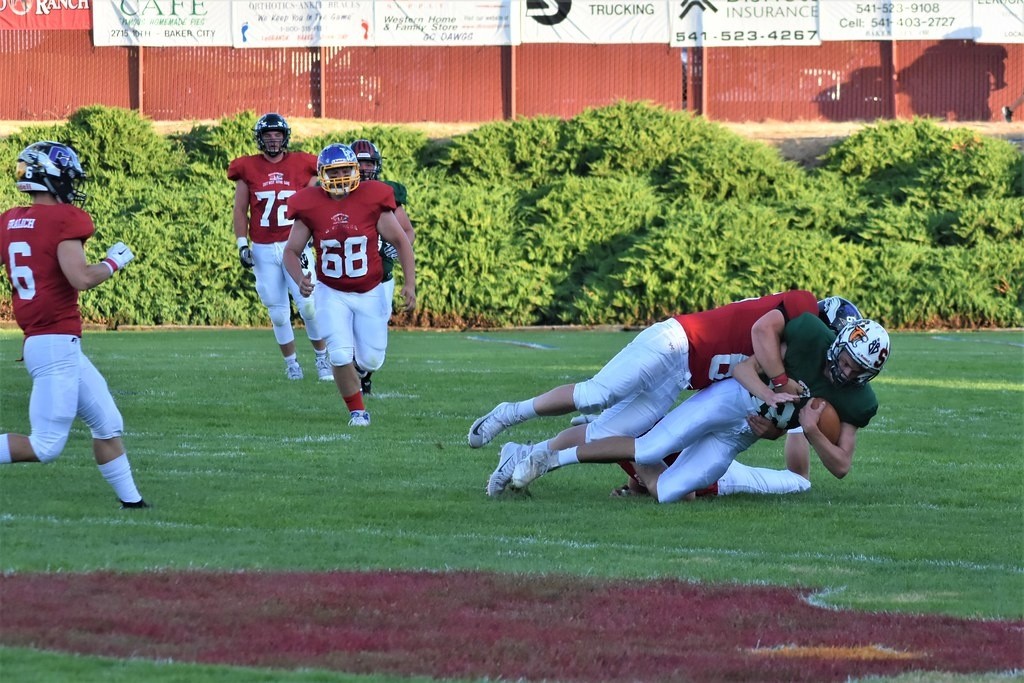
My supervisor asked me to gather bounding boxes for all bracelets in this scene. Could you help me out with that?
[770,371,788,387]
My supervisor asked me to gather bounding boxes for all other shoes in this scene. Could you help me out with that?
[119,498,149,508]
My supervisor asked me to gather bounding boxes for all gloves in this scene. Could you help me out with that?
[384,242,398,259]
[102,242,135,275]
[238,245,256,269]
[300,253,309,270]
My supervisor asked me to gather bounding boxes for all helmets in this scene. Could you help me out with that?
[316,143,360,194]
[16,141,86,203]
[255,113,290,151]
[827,318,891,384]
[817,296,864,337]
[350,140,382,173]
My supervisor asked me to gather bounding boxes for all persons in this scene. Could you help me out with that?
[284,139,418,425]
[0,139,149,508]
[469,291,891,502]
[226,114,335,382]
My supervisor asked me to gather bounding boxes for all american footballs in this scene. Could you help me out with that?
[803,397,841,447]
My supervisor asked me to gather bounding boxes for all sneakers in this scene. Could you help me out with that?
[571,414,599,426]
[285,358,304,380]
[512,449,562,488]
[348,409,371,427]
[315,350,334,381]
[468,402,524,448]
[353,349,368,378]
[485,441,534,497]
